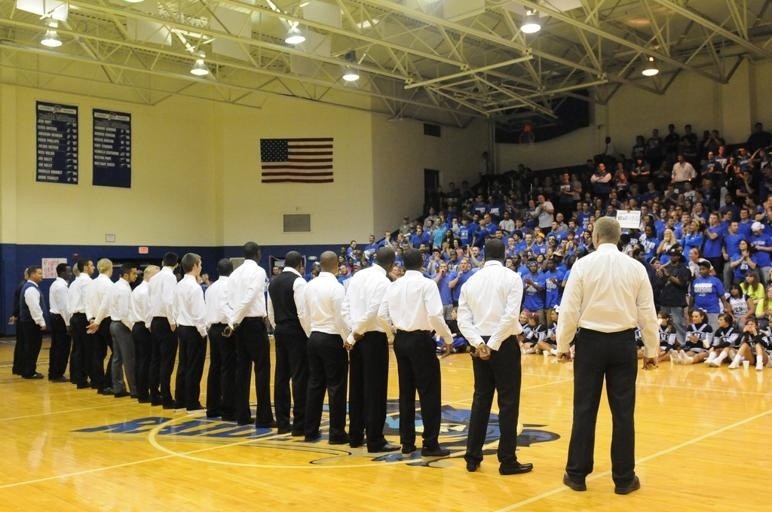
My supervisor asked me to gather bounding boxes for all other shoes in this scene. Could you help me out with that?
[50,375,66,382]
[238,418,254,424]
[77,383,91,388]
[368,443,400,452]
[306,430,321,442]
[291,427,305,435]
[278,423,293,434]
[255,420,278,429]
[99,384,237,421]
[329,434,350,444]
[23,372,44,378]
[350,440,367,448]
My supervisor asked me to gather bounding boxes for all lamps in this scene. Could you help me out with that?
[285,21,306,44]
[520,10,541,33]
[41,19,63,47]
[641,55,659,77]
[190,50,210,76]
[343,67,360,81]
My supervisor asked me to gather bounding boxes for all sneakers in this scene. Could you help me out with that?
[520,345,764,371]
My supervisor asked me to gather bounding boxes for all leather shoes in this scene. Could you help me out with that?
[466,461,480,472]
[563,473,586,491]
[615,475,641,494]
[499,461,534,474]
[402,444,417,454]
[421,446,451,456]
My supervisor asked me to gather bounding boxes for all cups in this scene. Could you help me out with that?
[742,360,749,368]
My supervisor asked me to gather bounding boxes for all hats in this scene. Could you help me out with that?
[535,232,546,242]
[645,218,765,269]
